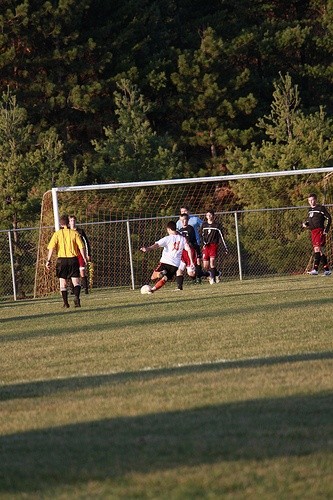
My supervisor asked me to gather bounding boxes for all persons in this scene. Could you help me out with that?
[177,213,201,290]
[200,209,228,285]
[175,206,210,281]
[69,216,91,295]
[46,216,87,308]
[303,194,332,275]
[140,221,195,292]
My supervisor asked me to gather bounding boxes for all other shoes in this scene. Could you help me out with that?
[191,280,196,286]
[148,289,154,294]
[324,271,332,276]
[85,289,89,295]
[197,278,202,284]
[176,286,183,291]
[216,275,221,284]
[73,299,81,307]
[64,303,70,308]
[210,279,215,285]
[159,270,167,278]
[307,269,318,275]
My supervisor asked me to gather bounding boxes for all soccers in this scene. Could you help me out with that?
[141,285,152,294]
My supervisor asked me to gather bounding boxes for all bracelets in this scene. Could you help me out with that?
[47,260,51,262]
